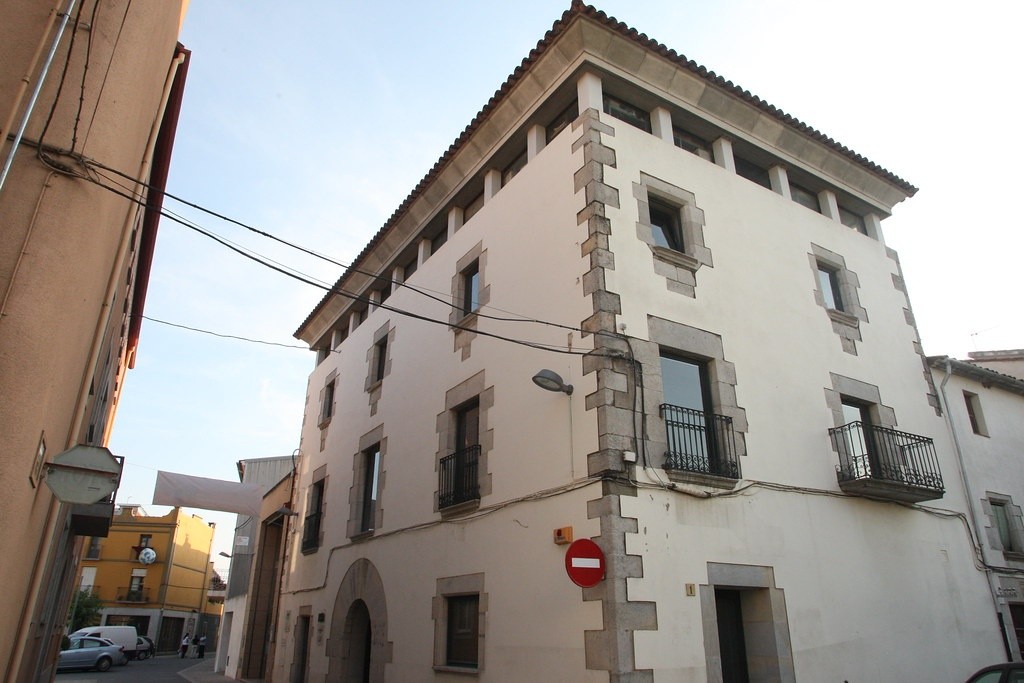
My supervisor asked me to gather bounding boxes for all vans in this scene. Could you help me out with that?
[66,625,138,660]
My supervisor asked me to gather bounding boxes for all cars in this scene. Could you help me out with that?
[56,636,125,673]
[127,635,156,661]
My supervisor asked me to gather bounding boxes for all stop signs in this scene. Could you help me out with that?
[565,538,606,588]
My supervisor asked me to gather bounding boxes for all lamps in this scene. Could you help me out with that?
[532,370,573,395]
[278,508,298,516]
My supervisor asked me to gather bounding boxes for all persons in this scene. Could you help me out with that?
[197,633,207,658]
[181,633,189,658]
[190,635,200,659]
